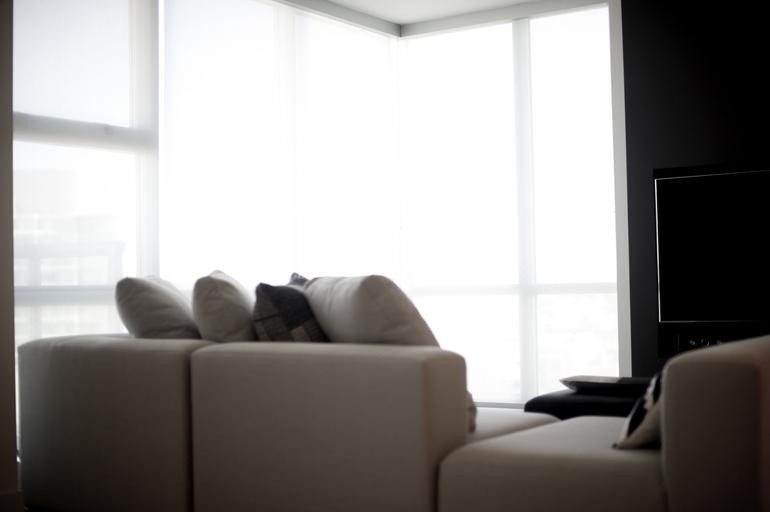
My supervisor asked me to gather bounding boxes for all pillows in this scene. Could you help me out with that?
[612,337,731,451]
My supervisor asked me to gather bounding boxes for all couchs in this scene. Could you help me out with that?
[18,332,770,512]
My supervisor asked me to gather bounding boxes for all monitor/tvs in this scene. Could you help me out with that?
[654,169,769,325]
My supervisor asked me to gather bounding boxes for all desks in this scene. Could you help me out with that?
[524,389,642,420]
